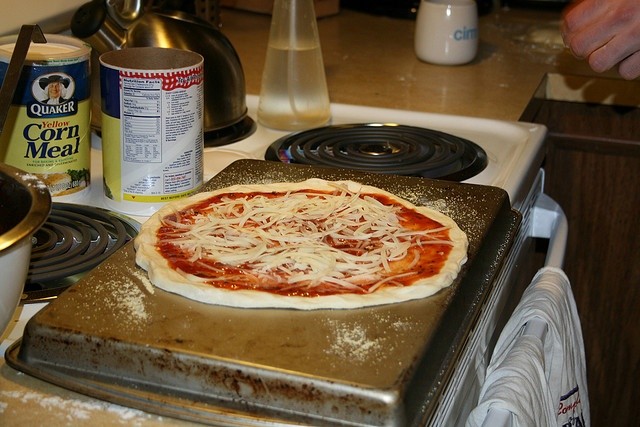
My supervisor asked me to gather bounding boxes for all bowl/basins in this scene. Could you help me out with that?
[1,163,50,341]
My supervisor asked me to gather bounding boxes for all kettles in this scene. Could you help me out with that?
[71,0,256,146]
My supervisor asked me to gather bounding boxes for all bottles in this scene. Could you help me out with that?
[257,2,332,129]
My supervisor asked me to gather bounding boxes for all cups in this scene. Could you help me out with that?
[413,2,481,64]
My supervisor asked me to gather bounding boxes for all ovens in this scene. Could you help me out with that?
[427,168,568,426]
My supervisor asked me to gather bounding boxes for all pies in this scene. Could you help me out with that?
[135,176,469,311]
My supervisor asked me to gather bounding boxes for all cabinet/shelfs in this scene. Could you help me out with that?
[1,3,639,427]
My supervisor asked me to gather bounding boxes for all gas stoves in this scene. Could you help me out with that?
[1,94,548,424]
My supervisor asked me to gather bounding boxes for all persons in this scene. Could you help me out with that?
[561,0,639,81]
